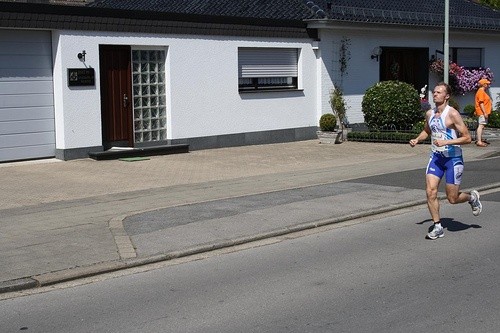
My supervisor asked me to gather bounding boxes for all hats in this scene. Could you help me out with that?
[478,79,491,85]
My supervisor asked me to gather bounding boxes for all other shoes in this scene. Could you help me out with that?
[476,142,487,147]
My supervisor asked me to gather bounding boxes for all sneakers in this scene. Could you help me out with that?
[468,190,482,216]
[426,226,445,240]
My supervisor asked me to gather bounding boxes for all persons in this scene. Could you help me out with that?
[474,79,492,146]
[409,82,482,240]
